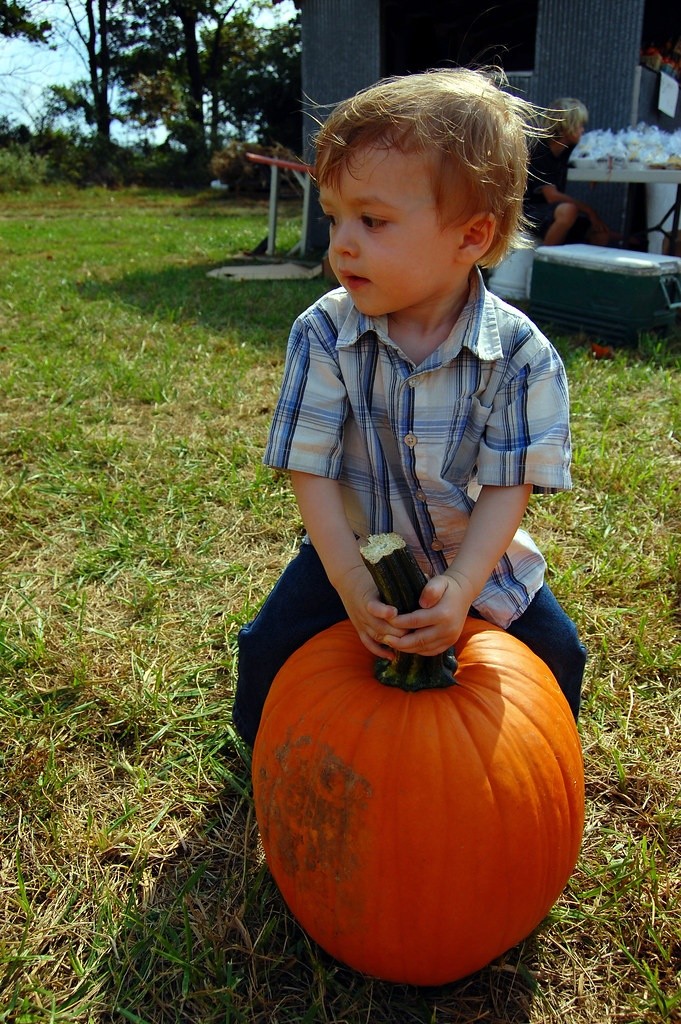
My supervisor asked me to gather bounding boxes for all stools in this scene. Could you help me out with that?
[478,229,545,301]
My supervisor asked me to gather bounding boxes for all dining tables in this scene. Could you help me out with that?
[567,162,681,256]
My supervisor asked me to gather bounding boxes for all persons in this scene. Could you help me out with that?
[231,68,588,750]
[523,98,610,247]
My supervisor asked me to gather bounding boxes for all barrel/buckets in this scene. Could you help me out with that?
[488,232,538,300]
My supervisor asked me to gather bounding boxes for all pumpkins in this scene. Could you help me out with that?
[591,343,610,358]
[253,531,586,986]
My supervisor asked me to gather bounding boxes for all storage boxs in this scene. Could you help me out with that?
[527,242,681,350]
